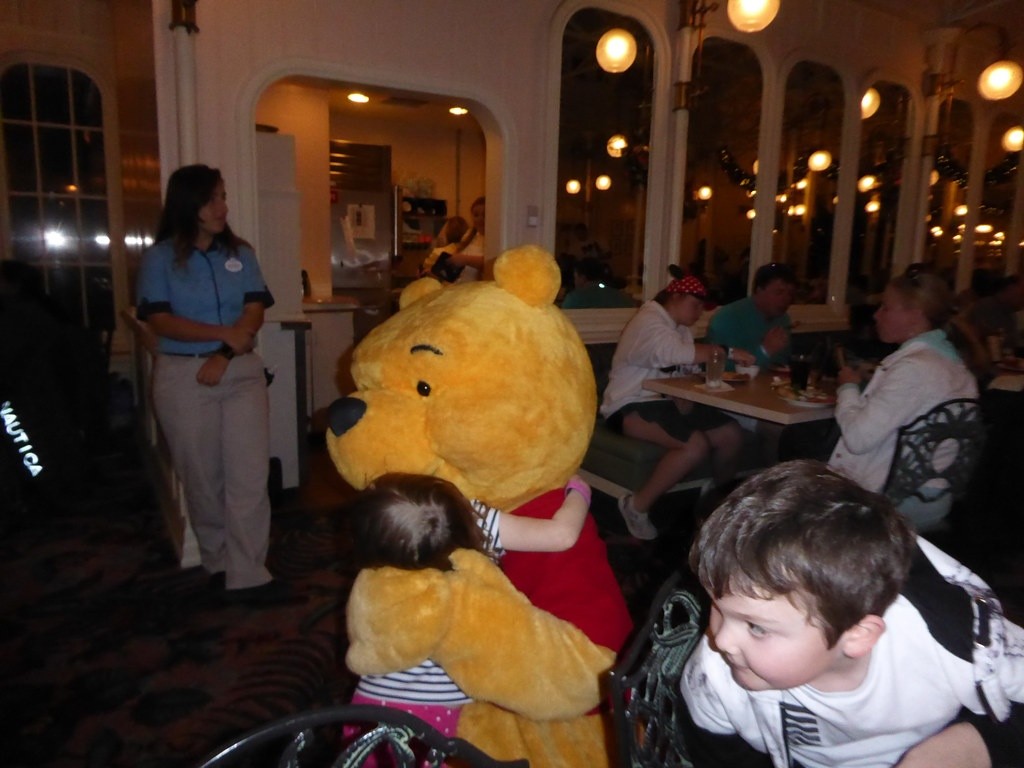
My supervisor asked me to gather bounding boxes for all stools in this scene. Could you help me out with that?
[581,414,768,510]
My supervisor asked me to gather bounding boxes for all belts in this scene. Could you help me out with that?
[167,350,216,358]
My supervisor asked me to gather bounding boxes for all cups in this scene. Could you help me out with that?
[790,354,814,391]
[706,350,724,389]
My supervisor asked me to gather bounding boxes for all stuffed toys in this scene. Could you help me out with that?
[326,245,647,768]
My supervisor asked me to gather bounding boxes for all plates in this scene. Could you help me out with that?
[695,371,750,382]
[997,363,1024,372]
[778,392,836,408]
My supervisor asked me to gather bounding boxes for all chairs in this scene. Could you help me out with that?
[609,563,774,768]
[202,704,528,768]
[884,399,992,550]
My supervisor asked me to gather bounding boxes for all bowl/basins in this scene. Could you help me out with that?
[735,364,760,379]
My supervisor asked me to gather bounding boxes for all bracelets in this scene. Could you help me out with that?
[218,344,235,359]
[565,480,592,509]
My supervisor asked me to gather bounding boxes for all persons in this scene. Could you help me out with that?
[554,242,890,309]
[330,472,593,768]
[597,257,1024,768]
[135,164,309,605]
[423,196,485,286]
[422,215,469,281]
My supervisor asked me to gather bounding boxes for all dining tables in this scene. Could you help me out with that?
[644,369,835,463]
[977,360,1024,394]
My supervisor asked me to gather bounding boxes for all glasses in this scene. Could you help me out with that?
[906,263,922,288]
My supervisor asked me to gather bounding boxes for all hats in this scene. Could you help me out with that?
[667,274,706,296]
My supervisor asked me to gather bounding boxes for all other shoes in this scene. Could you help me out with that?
[209,570,296,607]
[618,494,658,540]
